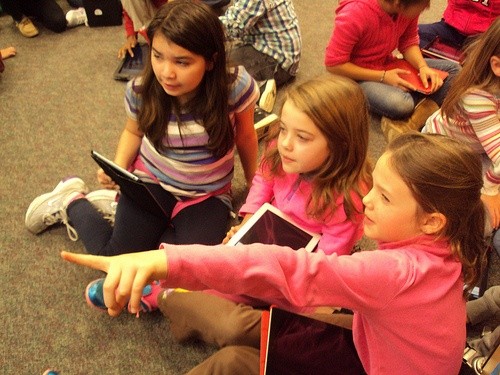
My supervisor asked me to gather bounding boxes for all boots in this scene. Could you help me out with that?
[381,98,440,145]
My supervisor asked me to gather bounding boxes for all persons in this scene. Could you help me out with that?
[25,0,303,256]
[325,0,500,239]
[0,0,122,77]
[61,75,500,375]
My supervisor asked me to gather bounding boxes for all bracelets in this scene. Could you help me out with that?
[380,70,386,83]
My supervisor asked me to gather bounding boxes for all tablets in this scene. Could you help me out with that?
[382,58,449,95]
[421,36,468,66]
[263,304,366,375]
[91,150,170,220]
[225,202,322,252]
[113,42,150,81]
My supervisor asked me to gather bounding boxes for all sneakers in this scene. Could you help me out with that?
[259,79,277,113]
[84,189,119,227]
[15,16,39,37]
[66,7,88,27]
[85,278,161,314]
[253,108,278,141]
[24,177,89,234]
